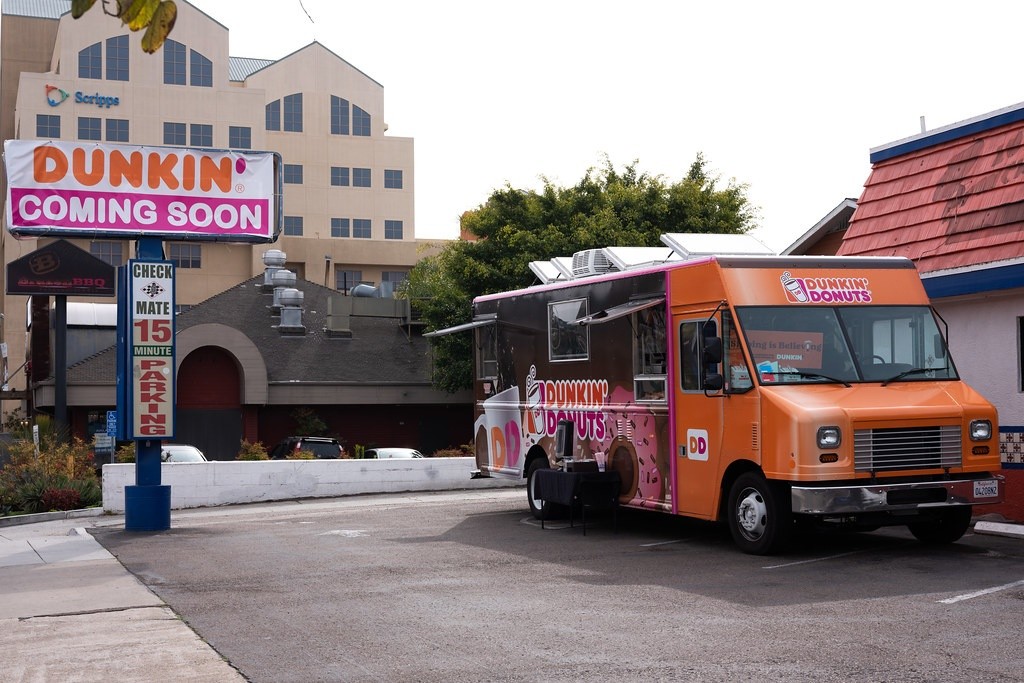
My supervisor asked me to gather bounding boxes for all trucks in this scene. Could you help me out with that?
[429,234,1005,551]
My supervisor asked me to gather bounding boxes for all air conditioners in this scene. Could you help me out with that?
[571,249,620,278]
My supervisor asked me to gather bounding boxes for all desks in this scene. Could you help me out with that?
[534,469,624,536]
